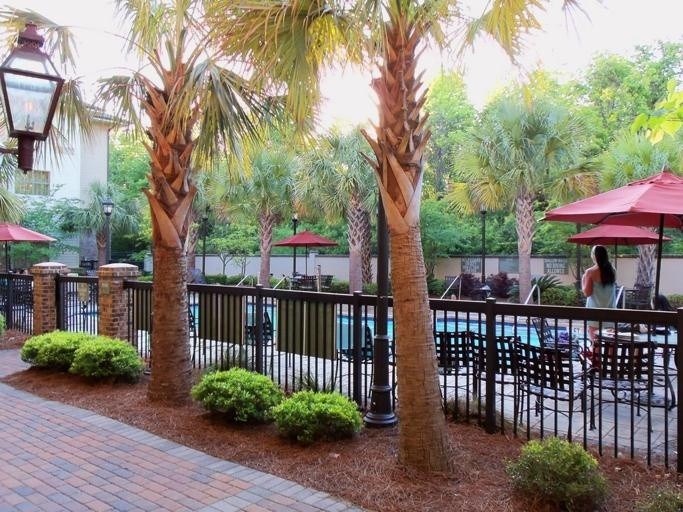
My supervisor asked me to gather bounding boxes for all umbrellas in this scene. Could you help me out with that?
[568,223,671,271]
[538,161,683,309]
[0,219,56,272]
[271,228,339,277]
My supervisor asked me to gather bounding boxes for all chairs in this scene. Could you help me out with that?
[615,280,654,311]
[290,273,333,291]
[442,275,460,300]
[327,313,680,438]
[185,306,207,358]
[225,310,291,374]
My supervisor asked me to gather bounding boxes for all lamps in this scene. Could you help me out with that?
[0,19,67,174]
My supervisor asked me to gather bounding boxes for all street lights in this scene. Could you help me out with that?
[0,14,65,181]
[99,192,119,267]
[286,202,300,283]
[197,197,213,277]
[475,185,495,293]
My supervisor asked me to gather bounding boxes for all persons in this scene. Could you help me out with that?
[639,295,677,336]
[582,245,617,370]
